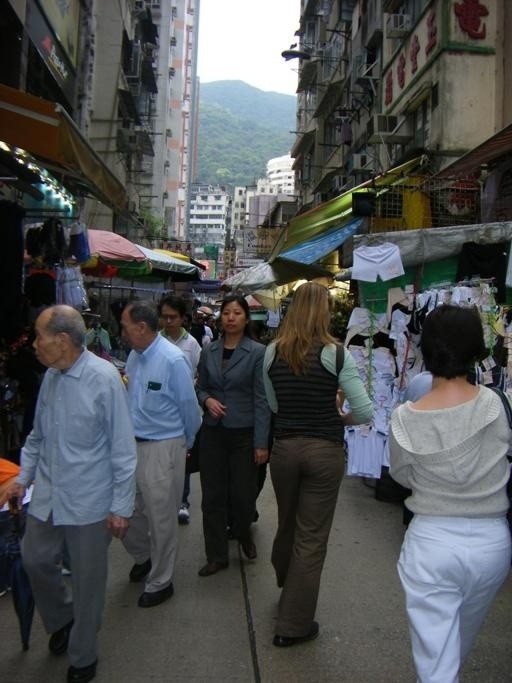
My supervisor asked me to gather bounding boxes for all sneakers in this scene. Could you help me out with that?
[177,501,190,525]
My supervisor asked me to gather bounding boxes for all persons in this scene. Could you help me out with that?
[263,283,373,647]
[196,295,268,577]
[120,299,203,608]
[8,305,136,682]
[388,303,512,683]
[150,296,205,522]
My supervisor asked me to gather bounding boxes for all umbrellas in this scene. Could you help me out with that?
[189,277,223,290]
[3,496,35,651]
[244,295,262,306]
[153,246,207,271]
[221,262,327,290]
[22,222,146,275]
[197,306,212,314]
[133,244,206,280]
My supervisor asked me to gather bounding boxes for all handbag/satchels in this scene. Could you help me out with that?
[491,386,512,534]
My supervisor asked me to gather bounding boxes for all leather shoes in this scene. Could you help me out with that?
[128,557,153,582]
[67,657,98,683]
[199,558,229,577]
[138,582,174,608]
[47,618,76,656]
[241,538,259,561]
[270,621,321,648]
